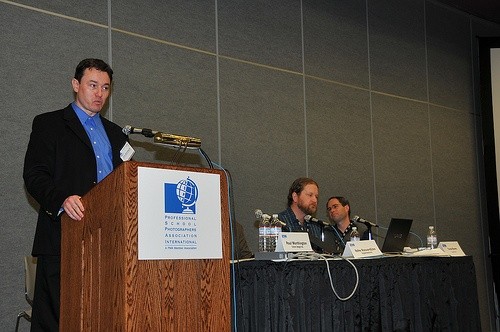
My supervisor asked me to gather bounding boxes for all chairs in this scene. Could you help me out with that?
[15,256,38,332]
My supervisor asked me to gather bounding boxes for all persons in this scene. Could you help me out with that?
[325,196,368,252]
[24,58,131,332]
[269,177,322,239]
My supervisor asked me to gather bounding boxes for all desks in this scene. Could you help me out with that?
[231,255,483,332]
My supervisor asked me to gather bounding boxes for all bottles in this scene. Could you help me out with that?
[259,214,282,253]
[427,225,438,250]
[350,227,360,241]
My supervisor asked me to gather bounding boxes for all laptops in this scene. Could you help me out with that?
[381,218,414,253]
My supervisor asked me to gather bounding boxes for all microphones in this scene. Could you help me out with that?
[353,215,378,228]
[254,210,287,227]
[304,215,329,226]
[122,125,164,138]
